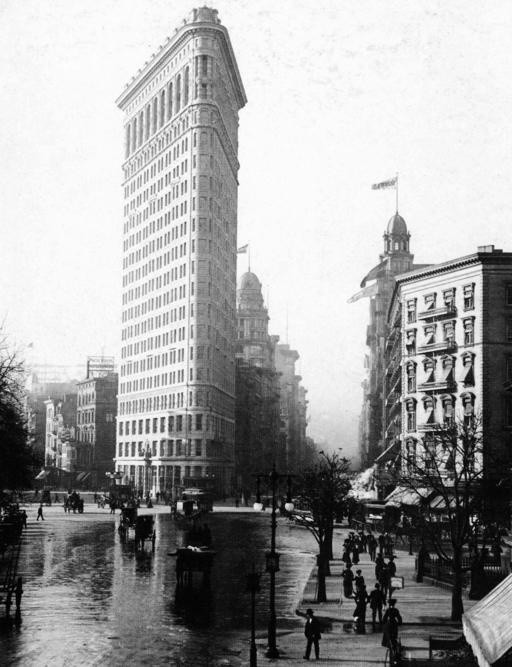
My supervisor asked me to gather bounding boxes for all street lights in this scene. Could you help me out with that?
[249,457,300,657]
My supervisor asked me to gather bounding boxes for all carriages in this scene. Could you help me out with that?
[118,506,156,552]
[63,488,83,513]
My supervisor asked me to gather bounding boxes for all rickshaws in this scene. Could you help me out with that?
[175,546,218,593]
[39,489,51,505]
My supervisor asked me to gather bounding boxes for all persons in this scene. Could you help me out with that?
[380,598,402,649]
[338,527,403,599]
[302,608,322,659]
[0,484,256,567]
[368,582,387,628]
[352,583,370,626]
